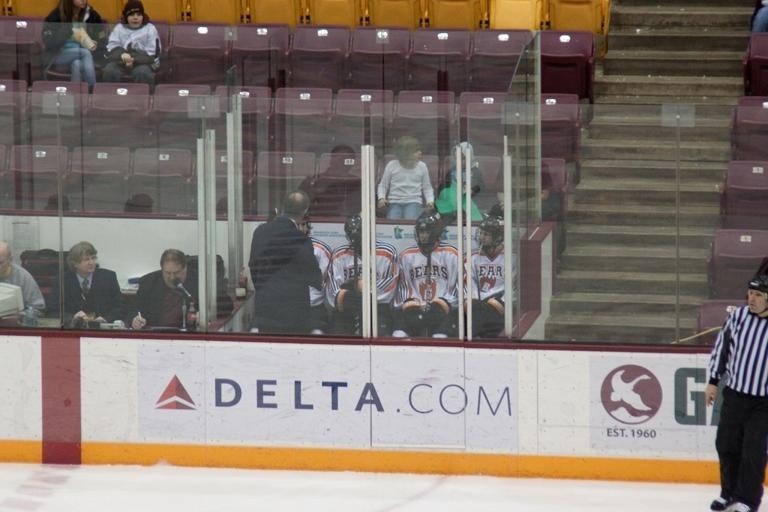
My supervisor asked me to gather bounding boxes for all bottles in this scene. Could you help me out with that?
[187,303,196,332]
[23,307,36,327]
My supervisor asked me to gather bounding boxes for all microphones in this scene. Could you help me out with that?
[175,279,192,299]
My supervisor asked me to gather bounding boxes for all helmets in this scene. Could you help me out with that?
[343,213,362,253]
[476,216,506,254]
[748,275,768,293]
[413,212,445,251]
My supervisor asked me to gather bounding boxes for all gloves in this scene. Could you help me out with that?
[403,296,422,326]
[335,279,362,313]
[483,296,505,325]
[425,297,450,325]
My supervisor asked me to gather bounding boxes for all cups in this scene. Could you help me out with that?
[70,28,95,50]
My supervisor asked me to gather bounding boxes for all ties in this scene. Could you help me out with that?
[81,277,90,297]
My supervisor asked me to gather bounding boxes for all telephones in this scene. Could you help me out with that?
[100,320,126,330]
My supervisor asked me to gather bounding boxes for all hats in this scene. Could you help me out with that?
[122,0,144,21]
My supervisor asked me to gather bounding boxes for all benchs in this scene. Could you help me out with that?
[0,210,552,341]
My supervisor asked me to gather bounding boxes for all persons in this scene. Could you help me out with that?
[705,275,768,512]
[751,0,768,32]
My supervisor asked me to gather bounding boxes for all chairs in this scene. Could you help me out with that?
[0,17,595,253]
[690,32,767,348]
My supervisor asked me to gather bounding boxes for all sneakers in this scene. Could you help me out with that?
[711,490,734,512]
[732,501,756,511]
